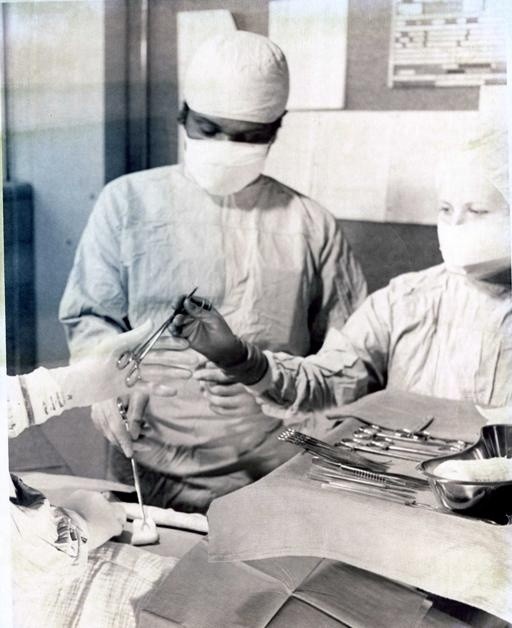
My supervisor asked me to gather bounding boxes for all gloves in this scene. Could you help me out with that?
[165,295,270,388]
[21,320,201,425]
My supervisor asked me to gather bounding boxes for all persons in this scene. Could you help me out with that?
[53,26,372,522]
[5,314,158,447]
[168,126,512,440]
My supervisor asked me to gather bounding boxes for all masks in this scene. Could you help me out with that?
[177,127,274,198]
[435,210,511,277]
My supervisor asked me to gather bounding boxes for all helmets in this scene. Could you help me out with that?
[182,30,292,124]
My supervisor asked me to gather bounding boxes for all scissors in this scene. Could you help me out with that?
[116,287,197,387]
[333,423,466,463]
[116,396,149,531]
